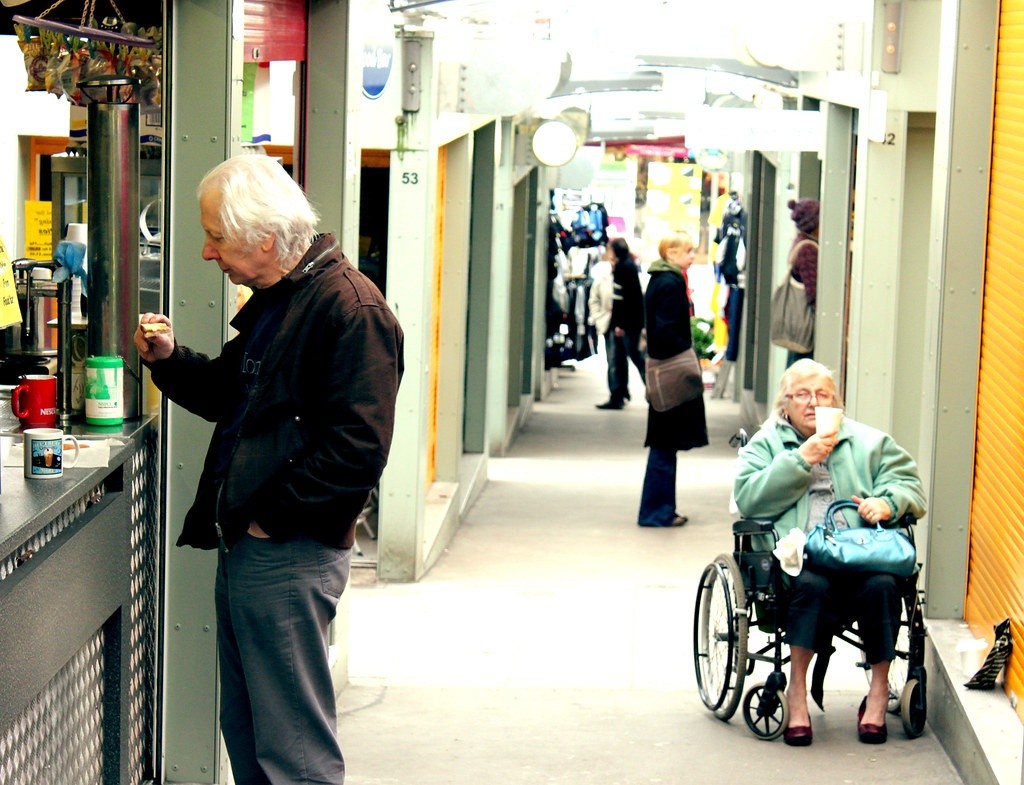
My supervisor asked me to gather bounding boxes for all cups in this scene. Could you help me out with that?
[11,374,57,424]
[815,407,842,447]
[66,223,87,311]
[24,428,80,479]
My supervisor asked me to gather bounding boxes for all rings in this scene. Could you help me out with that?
[870,512,874,516]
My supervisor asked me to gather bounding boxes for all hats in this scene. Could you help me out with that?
[788,200,819,232]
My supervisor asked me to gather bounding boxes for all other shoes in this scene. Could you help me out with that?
[783,713,813,746]
[672,518,684,526]
[857,696,887,744]
[596,403,622,409]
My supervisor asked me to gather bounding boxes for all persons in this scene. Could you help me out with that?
[132,154,403,784]
[783,199,820,368]
[732,358,927,748]
[587,242,630,403]
[595,237,647,410]
[551,192,748,367]
[638,233,709,528]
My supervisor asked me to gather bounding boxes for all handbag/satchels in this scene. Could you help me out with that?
[805,499,916,577]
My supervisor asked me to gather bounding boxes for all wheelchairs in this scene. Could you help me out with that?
[693,426,929,741]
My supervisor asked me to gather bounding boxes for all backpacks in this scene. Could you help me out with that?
[645,348,704,412]
[771,239,819,354]
